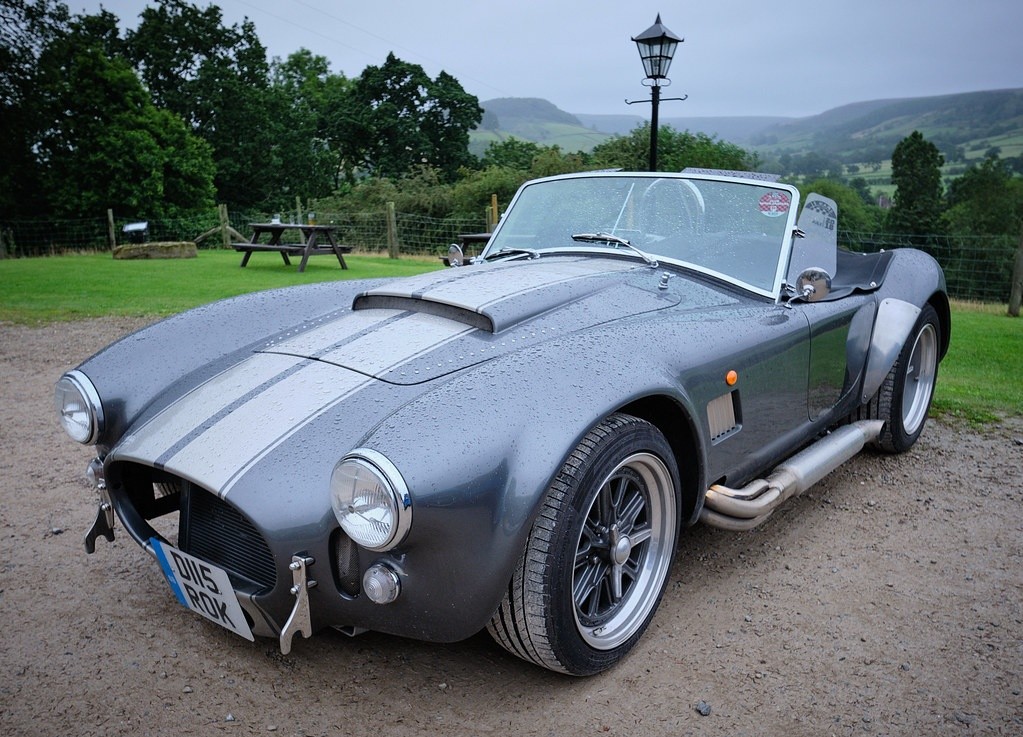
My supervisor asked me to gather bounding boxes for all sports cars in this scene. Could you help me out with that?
[57,169,950,675]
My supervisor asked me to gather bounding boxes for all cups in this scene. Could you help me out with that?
[290,215,294,224]
[307,213,317,225]
[298,215,303,224]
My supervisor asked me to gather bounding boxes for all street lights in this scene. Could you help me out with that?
[625,13,687,170]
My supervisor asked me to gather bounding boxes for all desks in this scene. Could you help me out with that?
[456,232,494,257]
[240,223,347,274]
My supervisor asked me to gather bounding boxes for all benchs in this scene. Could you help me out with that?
[438,256,476,267]
[229,243,302,252]
[289,243,352,255]
